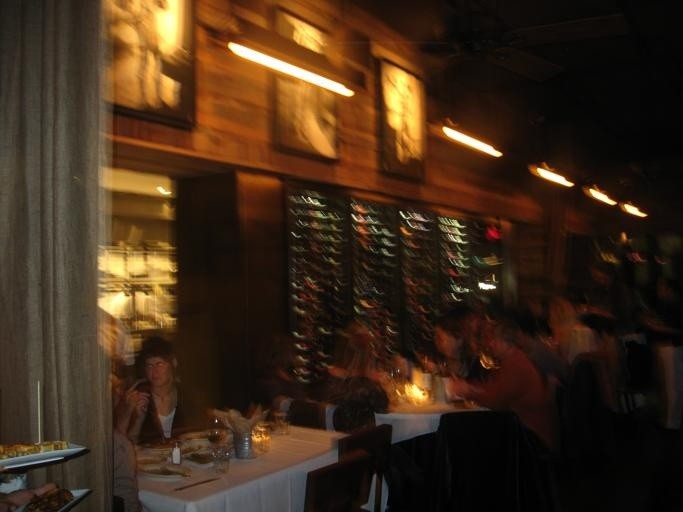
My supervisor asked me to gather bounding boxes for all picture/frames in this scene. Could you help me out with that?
[375,56,427,184]
[269,3,341,163]
[96,0,196,131]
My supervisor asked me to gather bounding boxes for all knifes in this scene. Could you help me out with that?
[175,473,222,492]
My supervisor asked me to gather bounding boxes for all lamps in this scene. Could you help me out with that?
[213,34,368,97]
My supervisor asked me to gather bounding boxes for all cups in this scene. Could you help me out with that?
[384,368,455,406]
[213,449,230,474]
[232,433,258,460]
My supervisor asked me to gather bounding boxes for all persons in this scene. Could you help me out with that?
[123,0,169,111]
[111,258,683,512]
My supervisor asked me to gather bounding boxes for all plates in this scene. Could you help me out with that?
[0,439,86,472]
[1,481,92,512]
[134,428,239,465]
[135,465,188,481]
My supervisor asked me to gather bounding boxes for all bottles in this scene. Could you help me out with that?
[251,410,292,450]
